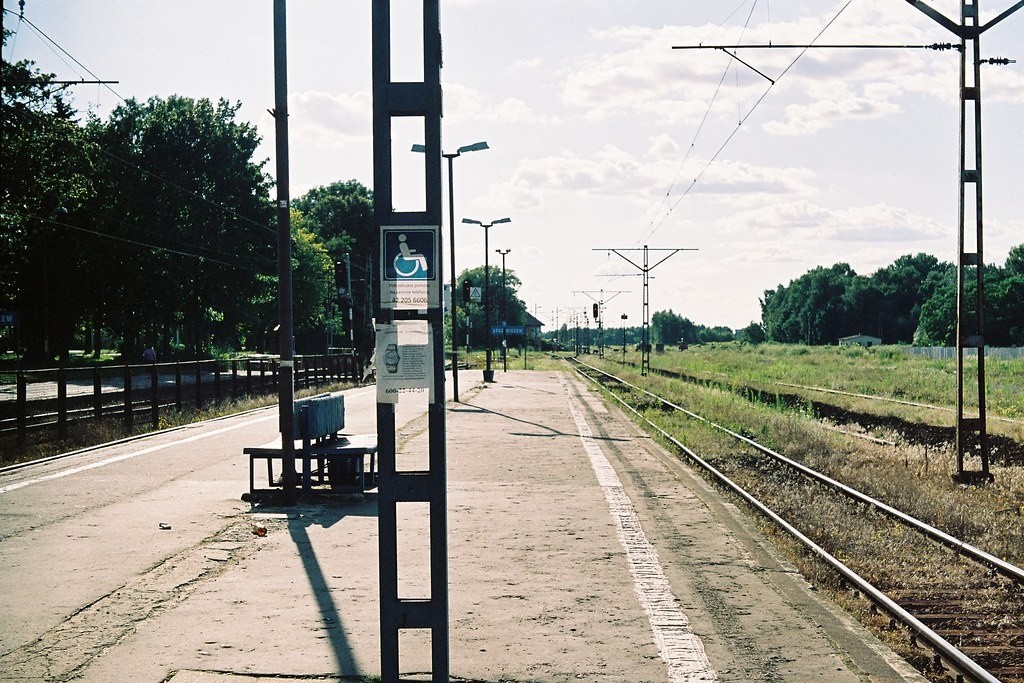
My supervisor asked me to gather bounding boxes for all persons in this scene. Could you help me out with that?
[143,344,157,380]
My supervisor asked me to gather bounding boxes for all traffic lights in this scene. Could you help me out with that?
[462,279,473,303]
[593,304,598,318]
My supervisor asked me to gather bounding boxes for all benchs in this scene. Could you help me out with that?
[244,392,332,493]
[306,394,377,493]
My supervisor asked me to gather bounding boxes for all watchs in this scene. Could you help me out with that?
[383,343,400,373]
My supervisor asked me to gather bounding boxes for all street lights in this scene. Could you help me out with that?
[462,218,512,383]
[496,249,511,372]
[411,141,490,402]
